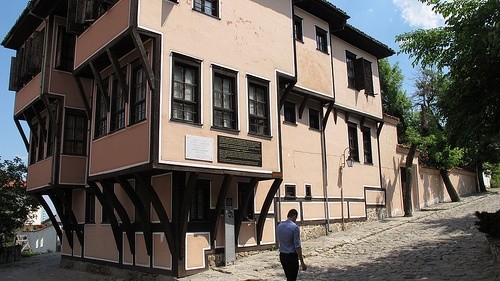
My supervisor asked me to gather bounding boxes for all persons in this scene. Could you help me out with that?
[276,209,307,281]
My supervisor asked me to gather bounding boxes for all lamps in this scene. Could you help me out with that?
[340,147,355,168]
